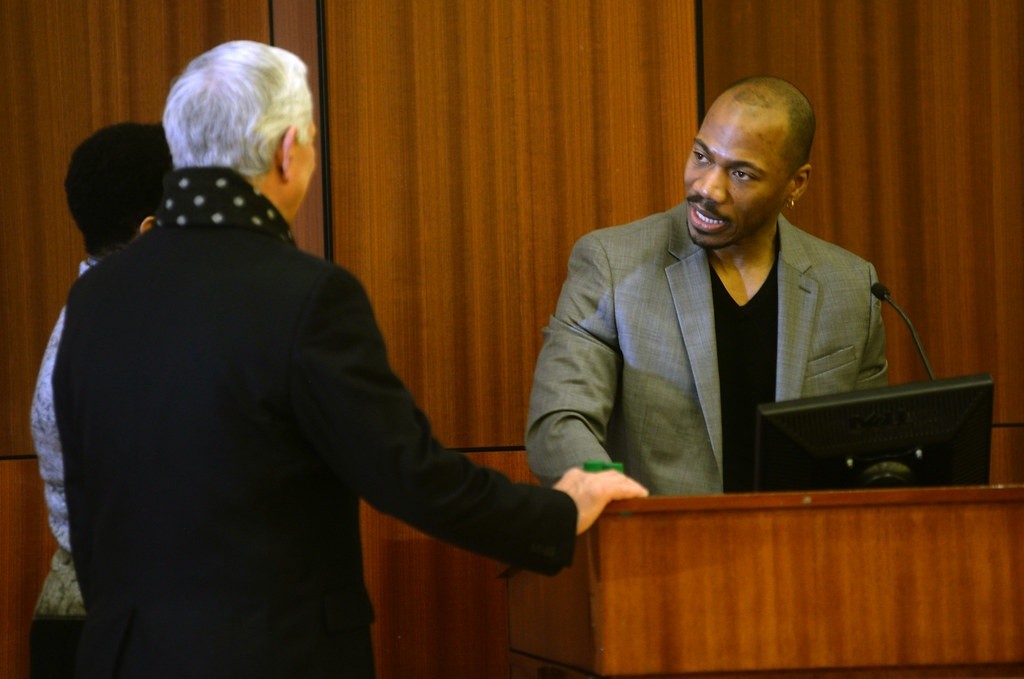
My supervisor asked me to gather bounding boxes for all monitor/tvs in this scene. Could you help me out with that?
[754,372,993,492]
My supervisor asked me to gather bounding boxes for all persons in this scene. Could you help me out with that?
[523,75,889,501]
[25,120,174,679]
[51,39,648,679]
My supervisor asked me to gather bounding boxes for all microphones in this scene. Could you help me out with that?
[871,283,935,381]
